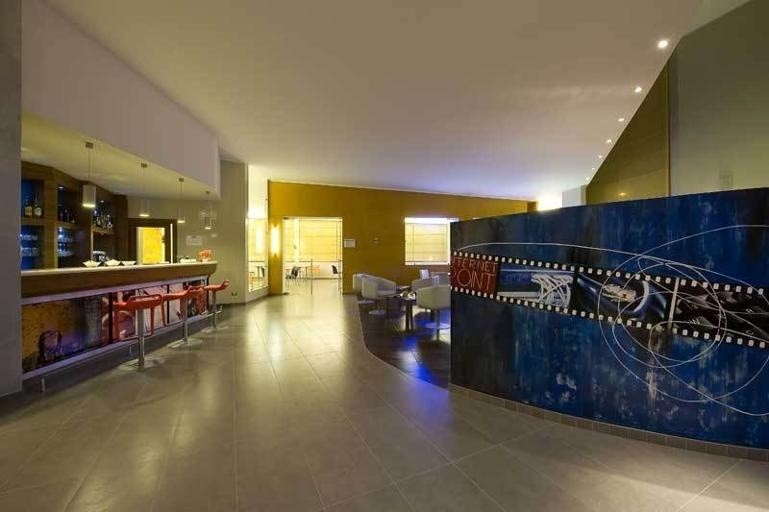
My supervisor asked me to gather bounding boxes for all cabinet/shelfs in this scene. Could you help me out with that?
[21,160,130,270]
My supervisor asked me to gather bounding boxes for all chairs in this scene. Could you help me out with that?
[331,265,342,281]
[353,269,450,331]
[286,266,299,287]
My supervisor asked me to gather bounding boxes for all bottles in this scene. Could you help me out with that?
[24,190,43,218]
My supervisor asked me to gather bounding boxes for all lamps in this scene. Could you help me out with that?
[81,143,97,209]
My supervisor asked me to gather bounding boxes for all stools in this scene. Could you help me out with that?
[112,279,231,372]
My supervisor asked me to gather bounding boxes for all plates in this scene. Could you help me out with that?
[83,260,136,267]
